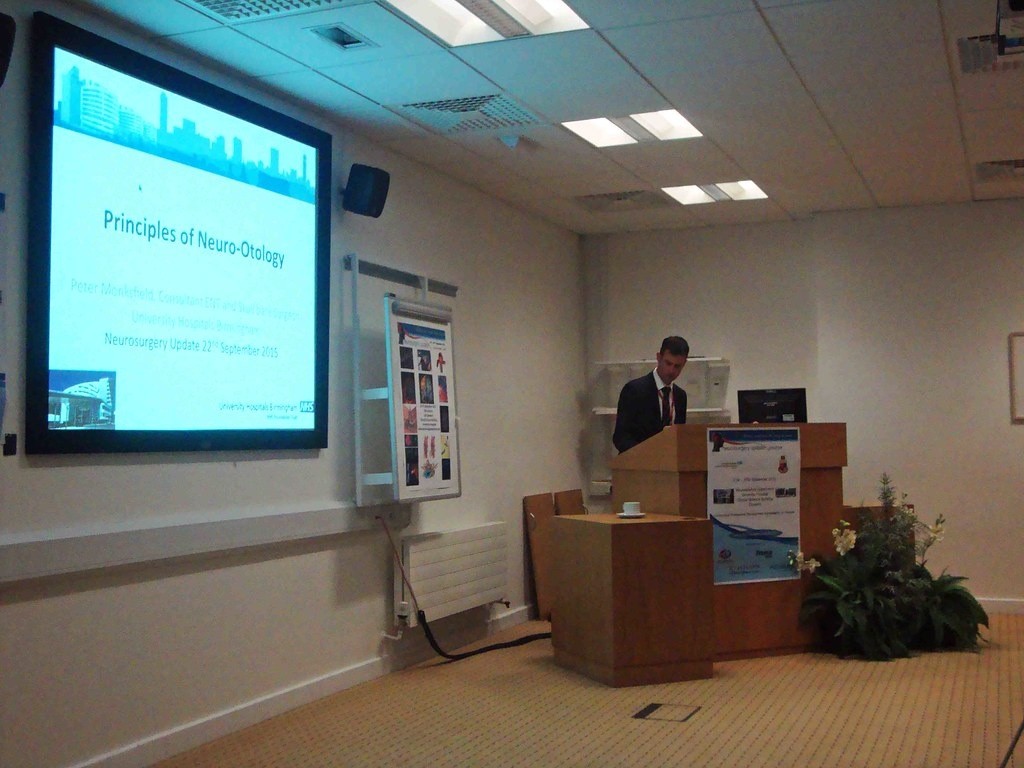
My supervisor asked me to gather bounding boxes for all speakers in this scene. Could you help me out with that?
[342,163,390,218]
[0,12,16,88]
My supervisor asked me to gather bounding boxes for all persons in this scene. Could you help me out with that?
[612,336,689,456]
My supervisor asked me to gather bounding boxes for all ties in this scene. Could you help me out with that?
[660,387,671,431]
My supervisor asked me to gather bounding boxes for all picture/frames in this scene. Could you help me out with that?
[1008,332,1024,423]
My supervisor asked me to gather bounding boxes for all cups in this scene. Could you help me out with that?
[623,502,640,515]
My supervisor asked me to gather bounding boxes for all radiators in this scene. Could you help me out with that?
[393,521,513,634]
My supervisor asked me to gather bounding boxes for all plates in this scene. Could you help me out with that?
[616,512,646,518]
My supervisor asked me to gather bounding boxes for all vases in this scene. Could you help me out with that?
[921,621,956,652]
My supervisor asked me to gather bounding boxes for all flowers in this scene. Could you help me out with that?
[786,472,990,661]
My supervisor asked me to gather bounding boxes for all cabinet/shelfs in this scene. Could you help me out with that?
[588,357,724,414]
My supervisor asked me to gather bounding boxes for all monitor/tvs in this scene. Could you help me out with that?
[737,388,808,426]
